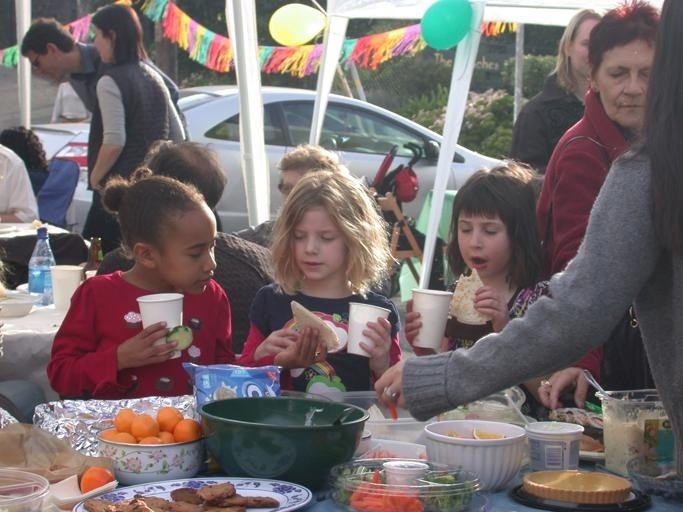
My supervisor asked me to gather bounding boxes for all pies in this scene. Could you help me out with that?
[449,268,494,326]
[291,300,340,353]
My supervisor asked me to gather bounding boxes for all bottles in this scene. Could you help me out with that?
[28,226,54,304]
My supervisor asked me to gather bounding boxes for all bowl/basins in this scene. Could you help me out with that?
[98,428,205,486]
[368,416,437,441]
[0,467,50,512]
[197,398,368,492]
[328,459,487,511]
[445,386,523,420]
[3,290,39,317]
[424,421,527,491]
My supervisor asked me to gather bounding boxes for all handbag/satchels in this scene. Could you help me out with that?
[597,302,651,392]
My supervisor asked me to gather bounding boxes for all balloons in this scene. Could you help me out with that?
[419,1,475,52]
[268,3,328,46]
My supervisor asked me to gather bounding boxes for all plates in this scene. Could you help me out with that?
[0,224,17,233]
[73,478,313,512]
[509,483,651,512]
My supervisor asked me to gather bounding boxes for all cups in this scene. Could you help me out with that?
[524,423,586,468]
[383,462,428,485]
[411,289,454,348]
[347,302,391,357]
[49,264,82,318]
[136,292,184,359]
[594,390,677,477]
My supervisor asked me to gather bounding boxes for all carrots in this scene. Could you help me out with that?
[348,468,425,512]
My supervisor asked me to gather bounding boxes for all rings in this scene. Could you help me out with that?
[539,379,553,387]
[384,386,399,401]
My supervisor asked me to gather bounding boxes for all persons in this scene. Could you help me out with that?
[84,4,185,253]
[375,0,683,510]
[238,170,403,390]
[0,125,49,195]
[511,8,605,177]
[94,139,276,357]
[535,2,660,411]
[47,177,329,402]
[402,161,553,422]
[230,144,349,250]
[0,145,42,289]
[23,17,190,144]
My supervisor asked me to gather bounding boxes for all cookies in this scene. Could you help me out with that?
[84,482,279,512]
[213,387,237,401]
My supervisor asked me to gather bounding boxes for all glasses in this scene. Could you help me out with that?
[276,175,294,194]
[31,44,48,67]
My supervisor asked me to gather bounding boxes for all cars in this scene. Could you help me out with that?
[30,83,517,233]
